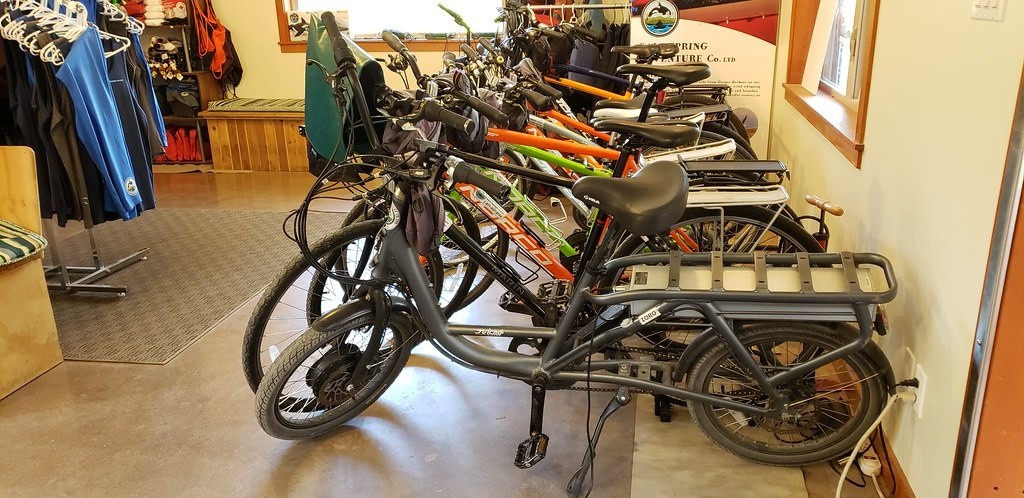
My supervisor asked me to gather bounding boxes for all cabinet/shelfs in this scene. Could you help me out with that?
[138,0,227,165]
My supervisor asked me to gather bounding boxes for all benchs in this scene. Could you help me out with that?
[0,144,66,401]
[198,98,316,173]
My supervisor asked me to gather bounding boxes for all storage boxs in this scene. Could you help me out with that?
[287,9,350,42]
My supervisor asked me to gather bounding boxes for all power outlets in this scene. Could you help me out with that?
[913,364,927,418]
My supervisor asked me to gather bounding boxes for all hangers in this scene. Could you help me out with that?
[548,2,630,28]
[0,0,146,66]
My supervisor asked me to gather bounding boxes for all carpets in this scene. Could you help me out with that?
[42,207,363,365]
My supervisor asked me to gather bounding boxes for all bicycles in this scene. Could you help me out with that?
[242,2,898,498]
